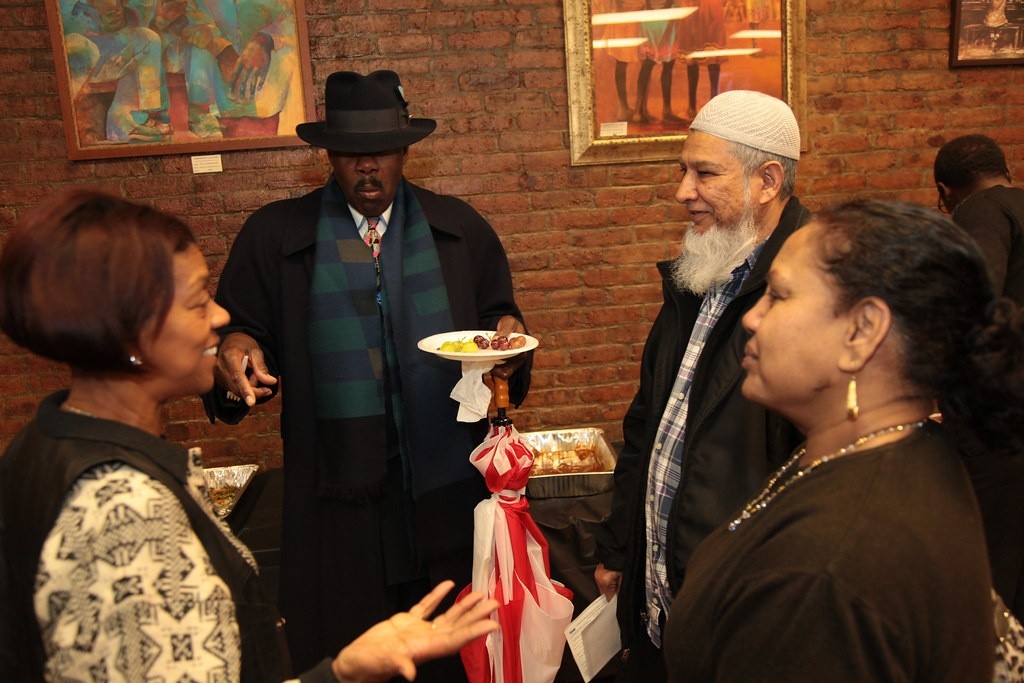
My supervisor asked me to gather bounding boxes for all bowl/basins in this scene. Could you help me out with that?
[516,427,618,500]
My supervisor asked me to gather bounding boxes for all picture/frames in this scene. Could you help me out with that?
[948,0,1024,69]
[564,0,809,166]
[43,0,314,161]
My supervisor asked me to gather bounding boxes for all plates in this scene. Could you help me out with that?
[418,331,539,362]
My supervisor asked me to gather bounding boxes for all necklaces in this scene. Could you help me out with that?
[728,422,925,531]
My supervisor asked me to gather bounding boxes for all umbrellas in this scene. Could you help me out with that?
[450,367,574,683]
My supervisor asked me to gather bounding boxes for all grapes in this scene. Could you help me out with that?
[441,335,526,354]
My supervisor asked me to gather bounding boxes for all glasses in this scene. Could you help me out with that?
[938,193,949,214]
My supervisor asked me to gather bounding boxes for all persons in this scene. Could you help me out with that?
[202,69,535,683]
[0,186,501,683]
[664,200,1024,683]
[594,90,812,683]
[934,133,1024,611]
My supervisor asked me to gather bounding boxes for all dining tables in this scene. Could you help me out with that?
[229,440,627,631]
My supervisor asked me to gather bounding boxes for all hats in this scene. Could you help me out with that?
[689,89,800,161]
[295,70,437,153]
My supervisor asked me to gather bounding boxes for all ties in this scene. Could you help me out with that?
[362,217,383,306]
